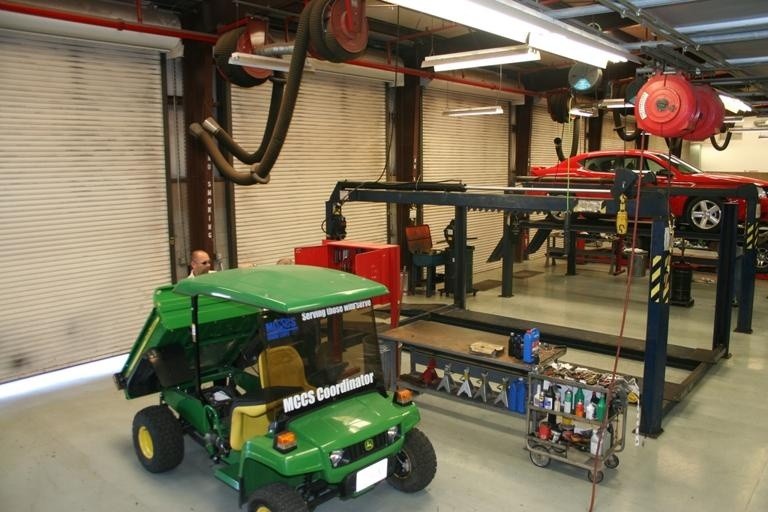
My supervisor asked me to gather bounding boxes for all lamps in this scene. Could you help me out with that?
[422,1,640,117]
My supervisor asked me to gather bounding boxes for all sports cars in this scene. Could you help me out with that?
[525,150,768,233]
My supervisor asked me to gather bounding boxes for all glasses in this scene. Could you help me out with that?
[195,261,212,265]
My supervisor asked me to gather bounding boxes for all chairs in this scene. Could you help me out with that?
[405,225,446,297]
[227,346,317,453]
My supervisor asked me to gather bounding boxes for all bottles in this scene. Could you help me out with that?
[508,327,541,365]
[589,427,606,456]
[534,381,608,422]
[507,376,526,414]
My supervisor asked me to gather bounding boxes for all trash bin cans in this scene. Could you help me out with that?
[438,247,477,297]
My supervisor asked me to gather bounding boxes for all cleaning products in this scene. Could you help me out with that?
[534,378,608,423]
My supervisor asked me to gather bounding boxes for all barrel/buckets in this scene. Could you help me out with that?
[507,377,518,411]
[523,327,540,363]
[515,334,524,358]
[589,427,612,456]
[508,332,516,355]
[518,376,530,414]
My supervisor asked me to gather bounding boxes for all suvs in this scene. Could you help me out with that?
[113,262,438,511]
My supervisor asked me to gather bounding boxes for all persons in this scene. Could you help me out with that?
[188,250,217,279]
